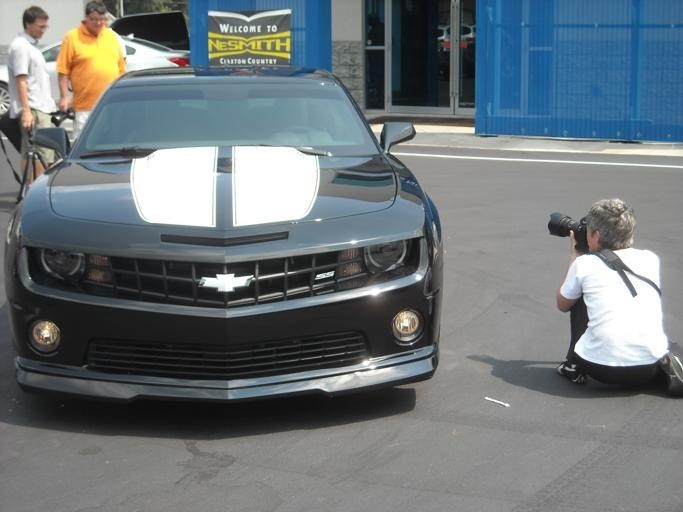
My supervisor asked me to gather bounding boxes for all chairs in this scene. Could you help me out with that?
[266,97,325,146]
[130,95,192,147]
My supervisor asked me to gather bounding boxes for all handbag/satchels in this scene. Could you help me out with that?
[0,108,23,185]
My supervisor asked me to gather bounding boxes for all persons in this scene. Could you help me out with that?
[56,0,127,142]
[8,6,59,184]
[556,198,683,396]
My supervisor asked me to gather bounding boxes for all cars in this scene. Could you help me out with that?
[3,65,444,404]
[0,29,190,117]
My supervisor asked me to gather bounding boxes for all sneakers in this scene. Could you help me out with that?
[556,361,587,384]
[656,352,683,396]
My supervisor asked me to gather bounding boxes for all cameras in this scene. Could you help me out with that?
[50,108,75,127]
[548,213,589,251]
